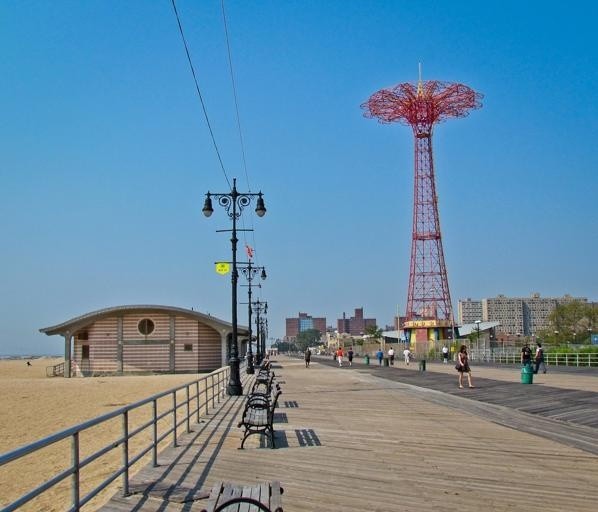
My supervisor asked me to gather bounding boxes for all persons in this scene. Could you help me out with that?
[389,346,395,364]
[532,344,547,374]
[347,345,354,366]
[402,347,411,366]
[441,343,449,363]
[337,347,344,367]
[455,344,475,388]
[520,341,533,365]
[305,346,312,369]
[377,348,383,366]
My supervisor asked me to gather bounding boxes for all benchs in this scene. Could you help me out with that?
[236,360,282,452]
[202,481,289,511]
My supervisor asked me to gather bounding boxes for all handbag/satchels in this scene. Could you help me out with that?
[454,362,462,371]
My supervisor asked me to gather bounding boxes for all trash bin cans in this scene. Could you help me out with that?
[384,358,388,366]
[364,356,369,365]
[419,360,425,371]
[521,367,533,384]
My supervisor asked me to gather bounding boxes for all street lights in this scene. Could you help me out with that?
[490,327,593,353]
[234,258,269,376]
[199,175,267,398]
[472,318,482,340]
